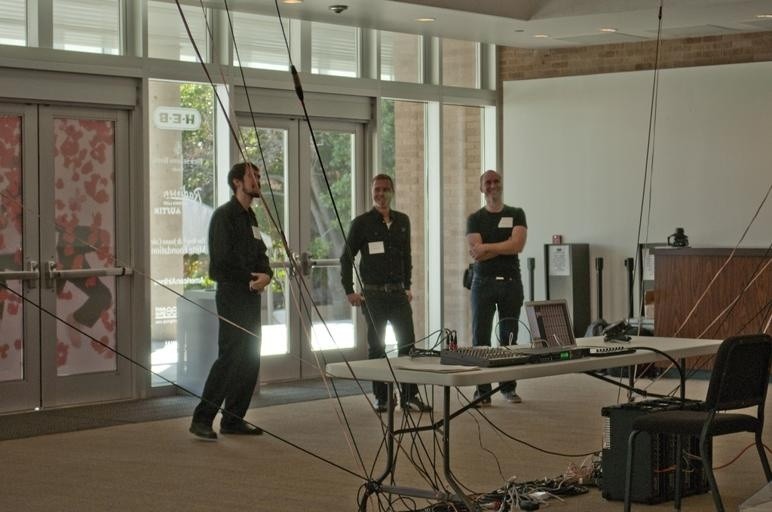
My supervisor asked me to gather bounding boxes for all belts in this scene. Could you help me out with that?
[364,282,405,293]
[475,268,520,280]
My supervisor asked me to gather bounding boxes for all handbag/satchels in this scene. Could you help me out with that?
[463,263,474,289]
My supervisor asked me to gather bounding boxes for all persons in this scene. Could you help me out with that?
[466,170,527,406]
[340,174,433,412]
[188,164,273,441]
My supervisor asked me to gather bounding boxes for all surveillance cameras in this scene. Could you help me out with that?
[329,5,347,14]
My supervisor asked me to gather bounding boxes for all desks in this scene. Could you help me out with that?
[326,335,723,512]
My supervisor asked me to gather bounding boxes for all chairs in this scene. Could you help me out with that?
[624,334,772,512]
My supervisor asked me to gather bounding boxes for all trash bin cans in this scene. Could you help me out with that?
[177,292,220,397]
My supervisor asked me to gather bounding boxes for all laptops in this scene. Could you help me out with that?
[525,299,636,357]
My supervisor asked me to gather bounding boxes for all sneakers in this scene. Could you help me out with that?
[401,398,432,412]
[502,390,522,402]
[476,399,491,406]
[373,393,397,412]
[188,418,263,442]
